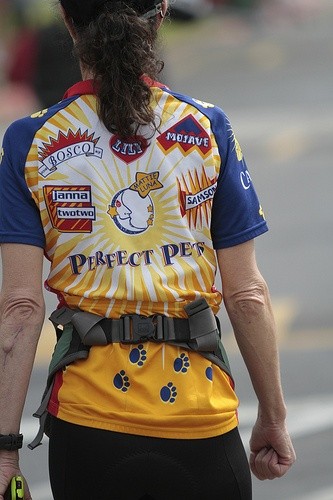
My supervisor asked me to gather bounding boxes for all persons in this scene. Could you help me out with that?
[0,0,297,500]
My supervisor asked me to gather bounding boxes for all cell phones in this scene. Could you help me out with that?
[10,476,24,500]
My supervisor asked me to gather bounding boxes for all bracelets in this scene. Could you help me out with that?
[0,430,24,452]
